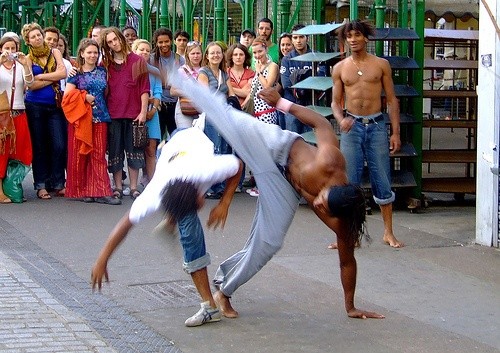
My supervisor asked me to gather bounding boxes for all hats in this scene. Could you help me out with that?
[1,32,19,40]
[241,29,256,39]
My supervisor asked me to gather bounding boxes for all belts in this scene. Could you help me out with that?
[345,112,384,124]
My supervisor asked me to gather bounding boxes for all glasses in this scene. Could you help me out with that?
[186,40,202,46]
[280,32,291,37]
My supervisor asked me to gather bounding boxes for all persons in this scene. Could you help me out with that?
[0,18,327,204]
[166,54,386,322]
[88,126,247,328]
[327,17,405,249]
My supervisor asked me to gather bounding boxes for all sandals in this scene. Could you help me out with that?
[53,186,66,196]
[130,189,141,199]
[112,188,123,199]
[37,189,51,199]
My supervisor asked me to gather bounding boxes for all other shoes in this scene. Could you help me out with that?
[79,197,95,203]
[205,190,225,199]
[234,187,241,193]
[97,196,121,205]
[0,193,12,203]
[246,186,260,196]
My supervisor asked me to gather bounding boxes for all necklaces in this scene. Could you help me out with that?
[350,56,368,76]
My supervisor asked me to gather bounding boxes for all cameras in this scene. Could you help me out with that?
[8,53,19,59]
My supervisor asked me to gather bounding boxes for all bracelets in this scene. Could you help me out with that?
[24,72,32,77]
[256,71,263,77]
[25,79,33,84]
[152,105,157,112]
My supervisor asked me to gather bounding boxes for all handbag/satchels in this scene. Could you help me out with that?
[132,122,150,148]
[0,110,17,156]
[178,66,204,115]
[2,158,32,203]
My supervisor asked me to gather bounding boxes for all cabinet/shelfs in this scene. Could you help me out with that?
[287,22,479,212]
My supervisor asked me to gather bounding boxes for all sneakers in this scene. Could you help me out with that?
[184,300,222,326]
